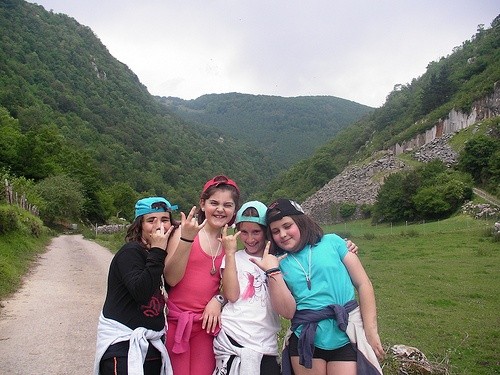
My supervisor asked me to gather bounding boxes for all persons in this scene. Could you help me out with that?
[217,201,358,375]
[249,199,385,375]
[163,175,240,375]
[94,197,179,375]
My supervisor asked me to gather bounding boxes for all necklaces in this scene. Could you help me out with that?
[290,244,311,290]
[202,227,221,275]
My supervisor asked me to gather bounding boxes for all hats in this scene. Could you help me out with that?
[266,199,304,224]
[134,195,178,219]
[235,201,269,226]
[204,174,240,194]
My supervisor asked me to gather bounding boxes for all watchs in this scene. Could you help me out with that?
[214,295,224,307]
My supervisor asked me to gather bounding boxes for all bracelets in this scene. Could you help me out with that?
[264,267,281,280]
[180,237,194,242]
